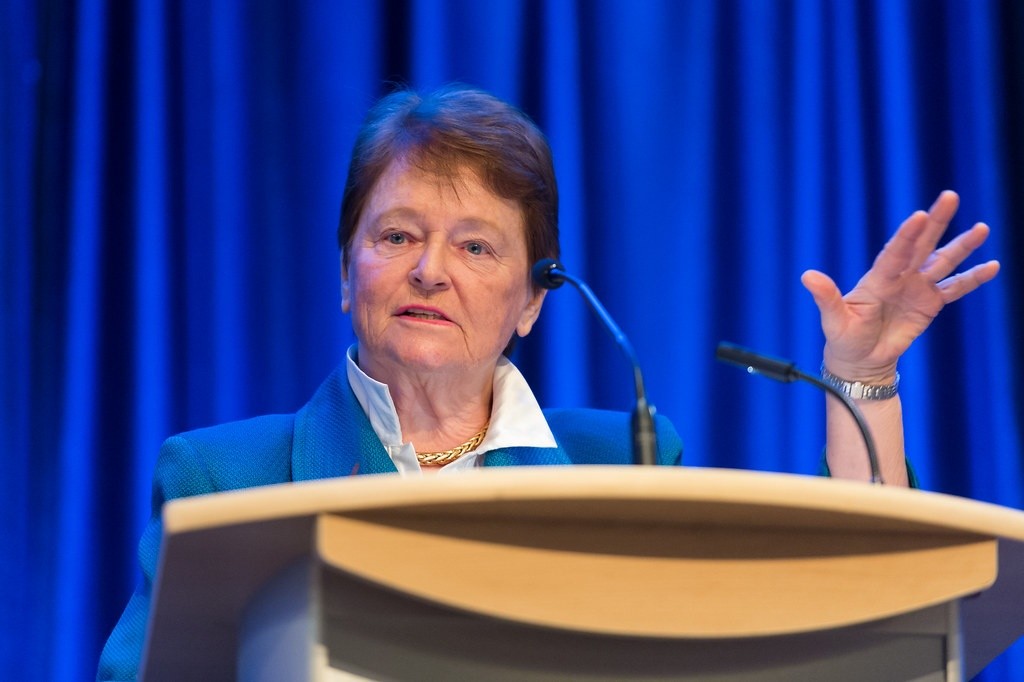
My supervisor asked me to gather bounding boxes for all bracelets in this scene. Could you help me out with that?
[820,362,900,400]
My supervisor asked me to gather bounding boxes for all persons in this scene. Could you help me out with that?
[94,83,999,682]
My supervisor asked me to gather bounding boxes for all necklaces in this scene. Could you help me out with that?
[416,421,490,466]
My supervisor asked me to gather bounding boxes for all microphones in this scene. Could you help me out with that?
[531,258,660,466]
[717,342,884,485]
